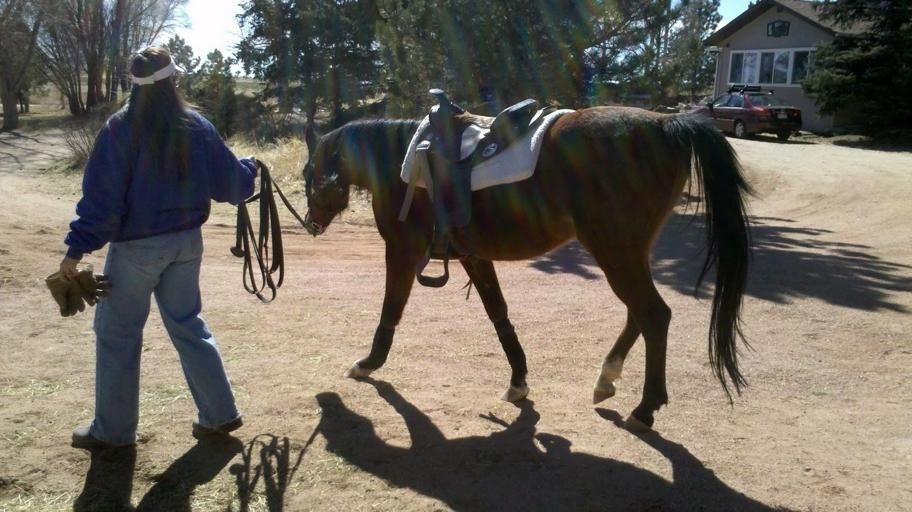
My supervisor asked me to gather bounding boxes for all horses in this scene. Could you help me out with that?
[299,105,767,435]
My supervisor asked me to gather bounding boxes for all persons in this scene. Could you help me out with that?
[60,47,261,447]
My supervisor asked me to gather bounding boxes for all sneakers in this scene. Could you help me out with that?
[72,428,135,449]
[192,414,244,435]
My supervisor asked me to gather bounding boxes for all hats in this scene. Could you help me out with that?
[132,57,184,86]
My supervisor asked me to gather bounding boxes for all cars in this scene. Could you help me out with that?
[689,85,802,140]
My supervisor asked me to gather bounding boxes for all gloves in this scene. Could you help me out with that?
[44,270,111,317]
